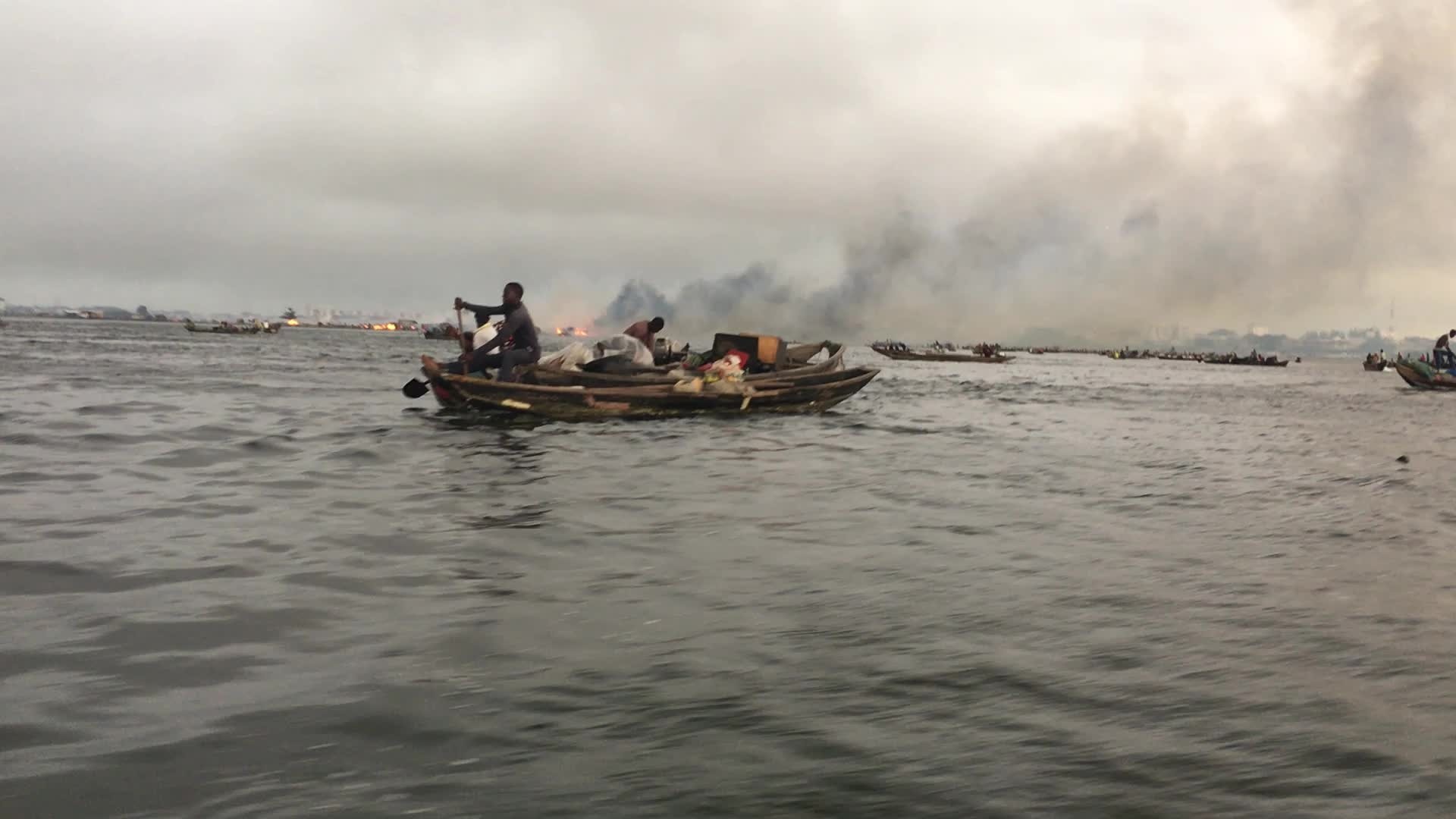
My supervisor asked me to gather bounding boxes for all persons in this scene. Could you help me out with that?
[1380,349,1386,363]
[1421,354,1426,361]
[422,314,501,375]
[1250,348,1264,361]
[624,317,664,353]
[454,281,541,383]
[584,334,653,376]
[885,338,906,350]
[1366,352,1379,364]
[1397,352,1403,359]
[1433,329,1456,371]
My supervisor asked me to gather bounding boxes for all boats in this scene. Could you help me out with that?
[1385,359,1398,368]
[867,340,1018,364]
[1101,345,1290,367]
[183,317,285,337]
[399,329,884,427]
[1362,360,1387,372]
[1395,352,1456,390]
[422,324,459,340]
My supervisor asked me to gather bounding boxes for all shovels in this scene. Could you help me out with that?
[402,378,431,400]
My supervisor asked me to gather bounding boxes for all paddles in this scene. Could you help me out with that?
[401,297,471,399]
